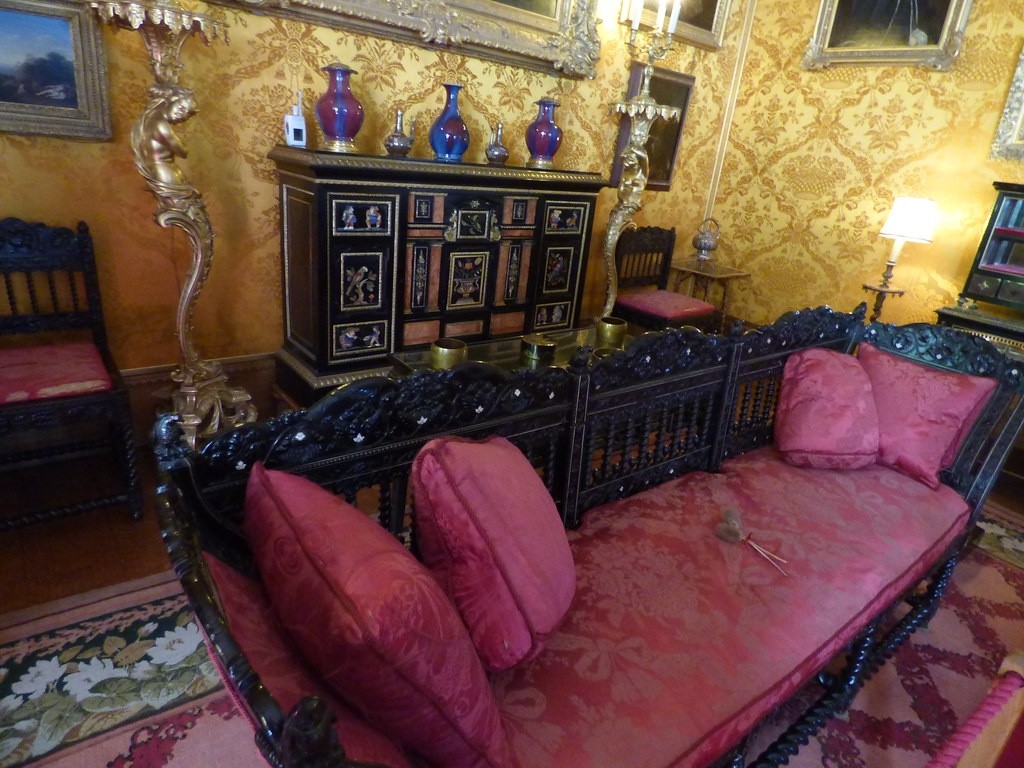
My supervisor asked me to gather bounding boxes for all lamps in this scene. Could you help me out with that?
[879,196,943,287]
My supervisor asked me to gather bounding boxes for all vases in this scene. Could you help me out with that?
[429,84,469,162]
[315,63,364,154]
[525,98,563,169]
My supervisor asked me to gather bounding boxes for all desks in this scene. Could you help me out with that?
[266,144,611,409]
[386,326,636,380]
[664,261,751,332]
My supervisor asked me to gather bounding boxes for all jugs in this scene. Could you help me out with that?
[692,218,721,250]
[485,121,509,165]
[384,109,416,156]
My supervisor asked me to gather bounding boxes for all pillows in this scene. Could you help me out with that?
[411,434,576,671]
[246,462,517,768]
[858,340,999,490]
[775,348,879,469]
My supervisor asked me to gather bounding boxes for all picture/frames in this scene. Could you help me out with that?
[200,0,602,81]
[0,0,112,140]
[610,63,695,192]
[988,45,1024,161]
[805,0,974,71]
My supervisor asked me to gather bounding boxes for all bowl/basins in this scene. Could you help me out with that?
[429,338,468,370]
[521,334,557,360]
[596,317,628,344]
[593,348,626,365]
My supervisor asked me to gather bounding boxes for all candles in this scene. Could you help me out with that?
[653,0,667,32]
[619,0,732,52]
[667,0,681,32]
[631,0,644,30]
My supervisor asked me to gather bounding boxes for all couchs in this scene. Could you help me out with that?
[154,302,1024,768]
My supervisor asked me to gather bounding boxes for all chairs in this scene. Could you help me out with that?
[604,225,715,334]
[0,217,144,530]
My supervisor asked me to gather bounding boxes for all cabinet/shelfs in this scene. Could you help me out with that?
[935,181,1024,502]
